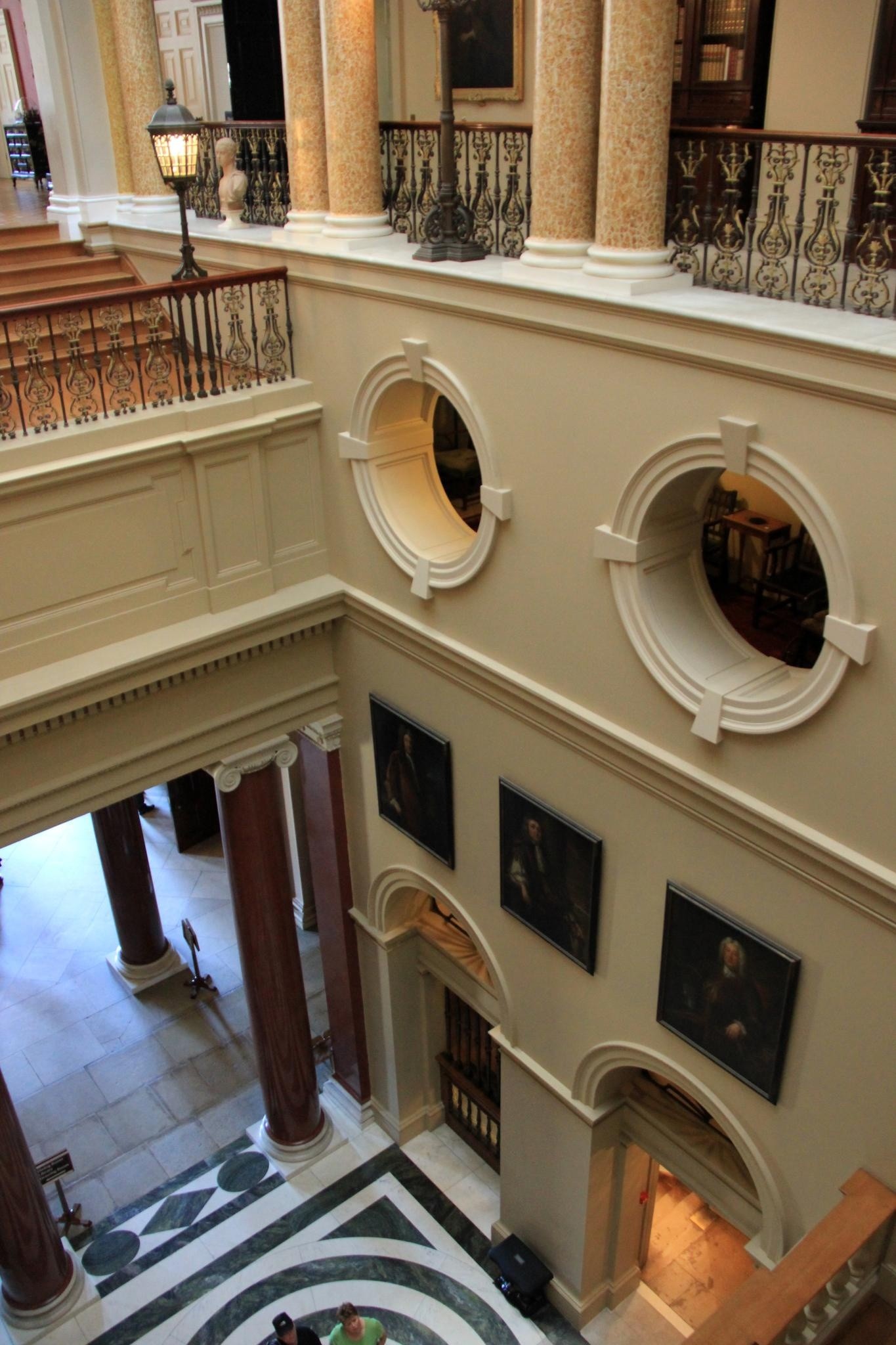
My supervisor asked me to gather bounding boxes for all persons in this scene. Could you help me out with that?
[329,1302,387,1345]
[134,791,154,815]
[215,137,247,211]
[786,609,828,665]
[267,1312,322,1345]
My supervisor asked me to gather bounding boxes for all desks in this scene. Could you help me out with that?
[715,508,792,608]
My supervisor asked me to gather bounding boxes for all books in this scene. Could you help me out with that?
[699,44,744,80]
[672,8,685,81]
[704,0,748,33]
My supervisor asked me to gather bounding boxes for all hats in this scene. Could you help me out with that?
[273,1312,294,1338]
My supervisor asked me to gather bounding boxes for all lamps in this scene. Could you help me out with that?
[145,79,204,194]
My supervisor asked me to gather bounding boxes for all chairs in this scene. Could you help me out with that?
[700,485,737,601]
[312,1029,335,1075]
[752,523,827,638]
[435,404,482,511]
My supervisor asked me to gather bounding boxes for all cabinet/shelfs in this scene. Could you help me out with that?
[666,0,762,244]
[841,1,895,272]
[3,120,50,190]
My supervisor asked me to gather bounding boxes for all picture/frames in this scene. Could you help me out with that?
[369,689,455,870]
[498,777,602,976]
[433,1,524,102]
[656,878,801,1108]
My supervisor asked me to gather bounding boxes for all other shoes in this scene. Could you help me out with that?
[139,804,155,815]
